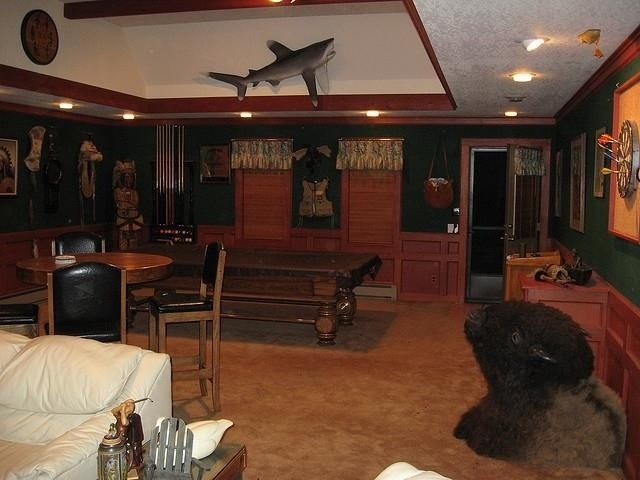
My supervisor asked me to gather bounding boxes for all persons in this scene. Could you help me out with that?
[111,399,145,466]
[111,159,144,249]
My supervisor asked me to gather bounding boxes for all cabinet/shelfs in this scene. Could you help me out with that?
[517,269,609,381]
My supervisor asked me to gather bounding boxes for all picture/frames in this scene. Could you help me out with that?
[21,9,60,66]
[198,142,232,184]
[606,71,640,245]
[568,132,587,235]
[1,137,19,197]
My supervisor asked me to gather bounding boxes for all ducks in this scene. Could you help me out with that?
[149,417,233,468]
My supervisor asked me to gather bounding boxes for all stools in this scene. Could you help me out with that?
[0,302,37,337]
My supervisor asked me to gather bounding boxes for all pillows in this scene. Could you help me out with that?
[0,332,142,416]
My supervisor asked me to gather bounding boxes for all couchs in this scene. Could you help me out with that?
[0,331,179,480]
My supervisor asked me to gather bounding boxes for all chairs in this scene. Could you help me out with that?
[46,230,129,342]
[146,239,227,412]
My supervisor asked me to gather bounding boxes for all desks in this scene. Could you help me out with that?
[125,243,381,346]
[15,253,176,337]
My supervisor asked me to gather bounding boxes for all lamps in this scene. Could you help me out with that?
[513,74,533,83]
[522,38,544,51]
